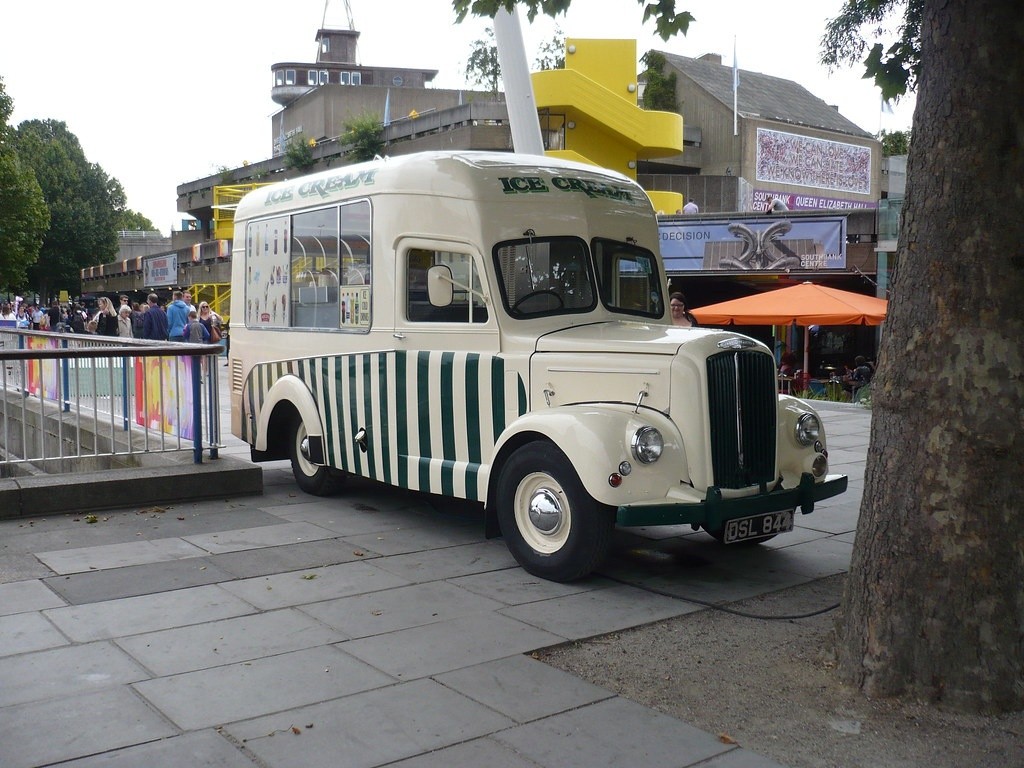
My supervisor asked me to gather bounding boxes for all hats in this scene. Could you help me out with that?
[59,291,69,302]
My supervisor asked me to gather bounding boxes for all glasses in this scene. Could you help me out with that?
[671,303,683,308]
[202,305,208,308]
[122,300,128,302]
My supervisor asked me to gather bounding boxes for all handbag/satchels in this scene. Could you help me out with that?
[211,325,221,343]
[22,320,30,326]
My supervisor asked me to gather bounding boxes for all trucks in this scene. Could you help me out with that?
[228,149,848,583]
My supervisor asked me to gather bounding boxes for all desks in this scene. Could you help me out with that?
[779,377,862,403]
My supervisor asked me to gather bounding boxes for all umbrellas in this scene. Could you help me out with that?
[686,280,890,398]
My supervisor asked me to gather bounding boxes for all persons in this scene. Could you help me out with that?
[59,302,91,334]
[0,300,61,332]
[224,318,230,367]
[89,290,222,384]
[765,197,789,212]
[683,199,699,214]
[776,351,874,397]
[670,292,699,327]
[676,210,681,215]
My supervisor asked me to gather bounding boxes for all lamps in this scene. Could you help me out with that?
[627,83,637,93]
[567,120,576,129]
[568,45,576,54]
[628,161,635,169]
